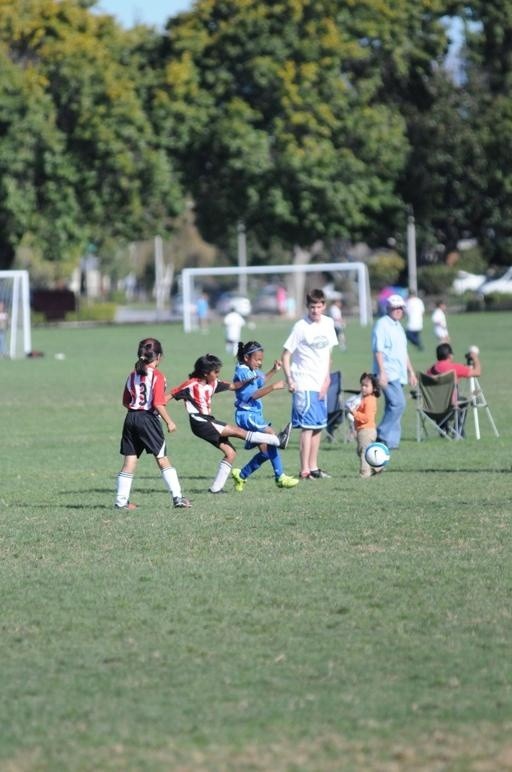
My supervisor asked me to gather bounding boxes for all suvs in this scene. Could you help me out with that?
[255,285,286,316]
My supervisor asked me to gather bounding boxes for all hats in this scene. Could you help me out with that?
[386,294,407,312]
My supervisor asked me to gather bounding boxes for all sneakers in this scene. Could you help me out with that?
[207,488,228,495]
[230,467,246,492]
[299,468,335,481]
[173,496,192,507]
[277,421,293,449]
[274,475,297,488]
[115,501,141,511]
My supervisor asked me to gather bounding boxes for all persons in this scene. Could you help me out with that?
[403,289,450,353]
[371,292,419,451]
[115,338,192,509]
[230,340,300,491]
[281,289,339,480]
[346,371,383,477]
[424,343,480,439]
[196,266,346,360]
[164,353,292,495]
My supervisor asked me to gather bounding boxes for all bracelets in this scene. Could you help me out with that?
[286,375,292,379]
[409,371,416,377]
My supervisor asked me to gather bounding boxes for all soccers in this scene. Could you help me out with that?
[365,441,391,467]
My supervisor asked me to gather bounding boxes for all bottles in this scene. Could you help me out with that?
[345,392,362,410]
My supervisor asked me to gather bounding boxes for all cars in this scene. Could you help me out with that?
[214,290,253,318]
[375,283,409,316]
[449,269,487,296]
[172,288,210,318]
[478,266,512,297]
[321,281,359,300]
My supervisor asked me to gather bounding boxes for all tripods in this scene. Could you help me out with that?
[461,371,498,439]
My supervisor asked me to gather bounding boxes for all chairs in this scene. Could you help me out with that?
[325,371,362,447]
[415,367,473,440]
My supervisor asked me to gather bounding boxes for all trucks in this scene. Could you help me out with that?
[1,282,78,324]
